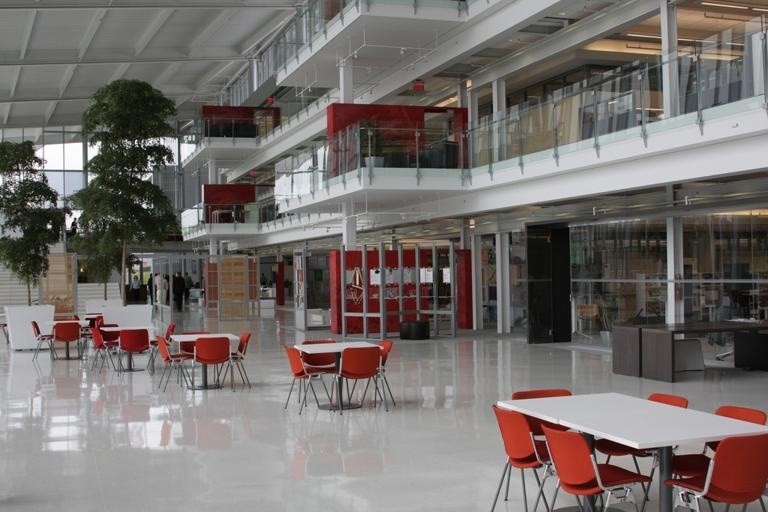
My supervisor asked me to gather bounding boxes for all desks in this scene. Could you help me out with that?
[294,340,384,415]
[642,321,767,384]
[45,320,90,360]
[212,210,249,224]
[611,322,668,377]
[99,325,146,372]
[496,392,767,512]
[169,333,240,390]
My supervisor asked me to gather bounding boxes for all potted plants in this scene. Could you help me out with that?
[62,77,182,328]
[0,140,73,350]
[365,124,384,168]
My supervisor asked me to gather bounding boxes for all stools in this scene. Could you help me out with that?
[577,303,600,345]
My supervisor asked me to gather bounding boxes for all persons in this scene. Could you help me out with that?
[172,271,184,312]
[156,274,169,305]
[148,273,153,306]
[71,217,79,236]
[184,271,194,306]
[260,272,267,285]
[129,275,142,303]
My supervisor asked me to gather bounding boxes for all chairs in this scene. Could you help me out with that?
[89,327,118,373]
[30,321,59,361]
[669,406,767,478]
[101,324,124,372]
[215,331,251,389]
[504,389,572,511]
[349,340,396,408]
[283,345,335,414]
[178,331,219,386]
[155,335,195,392]
[298,339,340,406]
[146,321,176,369]
[665,434,768,512]
[80,316,103,360]
[591,394,689,504]
[490,405,552,511]
[118,329,149,374]
[539,422,653,511]
[329,346,389,414]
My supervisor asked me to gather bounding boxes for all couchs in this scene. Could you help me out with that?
[400,320,430,339]
